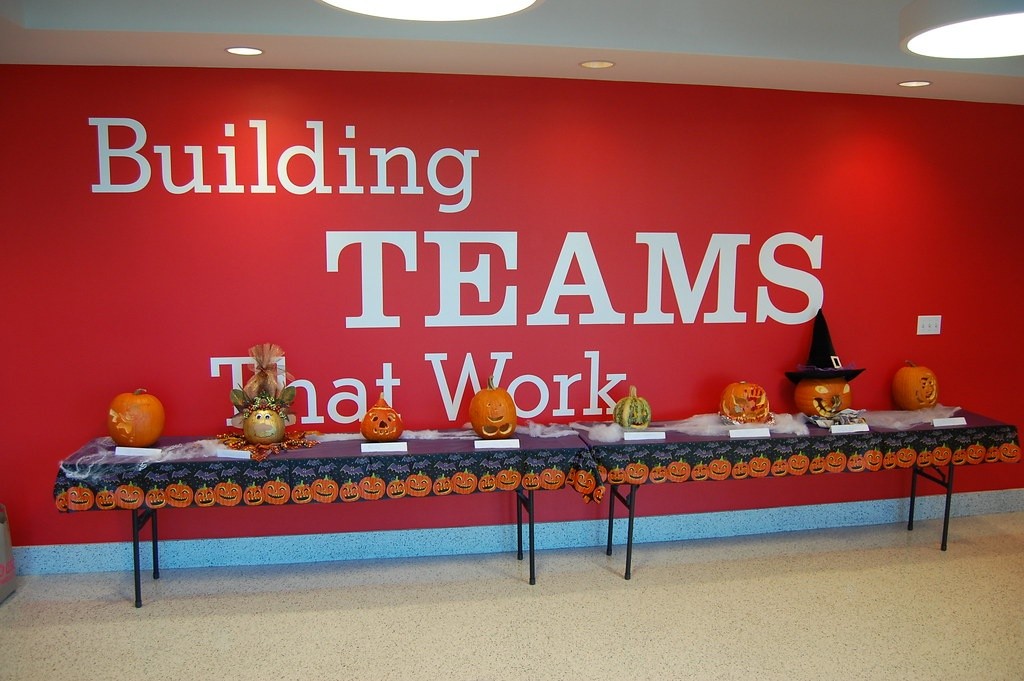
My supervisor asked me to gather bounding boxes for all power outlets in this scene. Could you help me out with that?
[917,315,942,335]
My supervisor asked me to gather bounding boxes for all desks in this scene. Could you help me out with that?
[53,423,606,609]
[571,404,1024,580]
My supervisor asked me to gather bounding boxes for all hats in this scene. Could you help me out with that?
[785,308,866,384]
